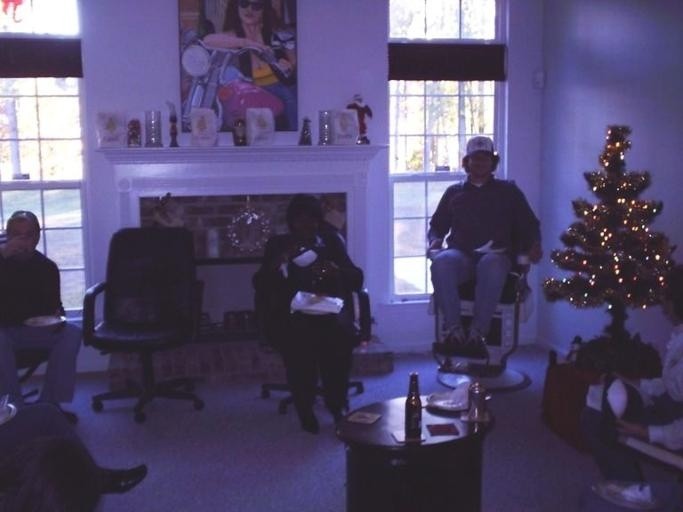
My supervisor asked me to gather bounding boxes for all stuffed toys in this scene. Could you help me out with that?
[343,91,374,138]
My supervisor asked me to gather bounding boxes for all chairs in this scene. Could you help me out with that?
[426,234,535,379]
[255,234,371,416]
[2,295,65,403]
[83,228,208,423]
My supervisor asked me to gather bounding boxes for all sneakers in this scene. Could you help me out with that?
[295,404,348,434]
[442,325,492,349]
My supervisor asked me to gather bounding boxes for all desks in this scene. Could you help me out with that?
[334,392,494,511]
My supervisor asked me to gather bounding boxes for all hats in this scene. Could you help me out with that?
[462,135,496,165]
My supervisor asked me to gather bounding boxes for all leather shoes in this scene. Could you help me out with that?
[81,465,150,495]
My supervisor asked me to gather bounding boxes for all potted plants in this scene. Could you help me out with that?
[538,125,677,454]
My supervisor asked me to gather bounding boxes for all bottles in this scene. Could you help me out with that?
[404,372,423,440]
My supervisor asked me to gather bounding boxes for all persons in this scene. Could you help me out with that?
[0,402,147,512]
[265,193,365,435]
[424,134,543,356]
[584,263,683,511]
[0,210,84,424]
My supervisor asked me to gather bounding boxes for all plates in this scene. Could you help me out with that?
[425,390,469,412]
[22,315,67,328]
